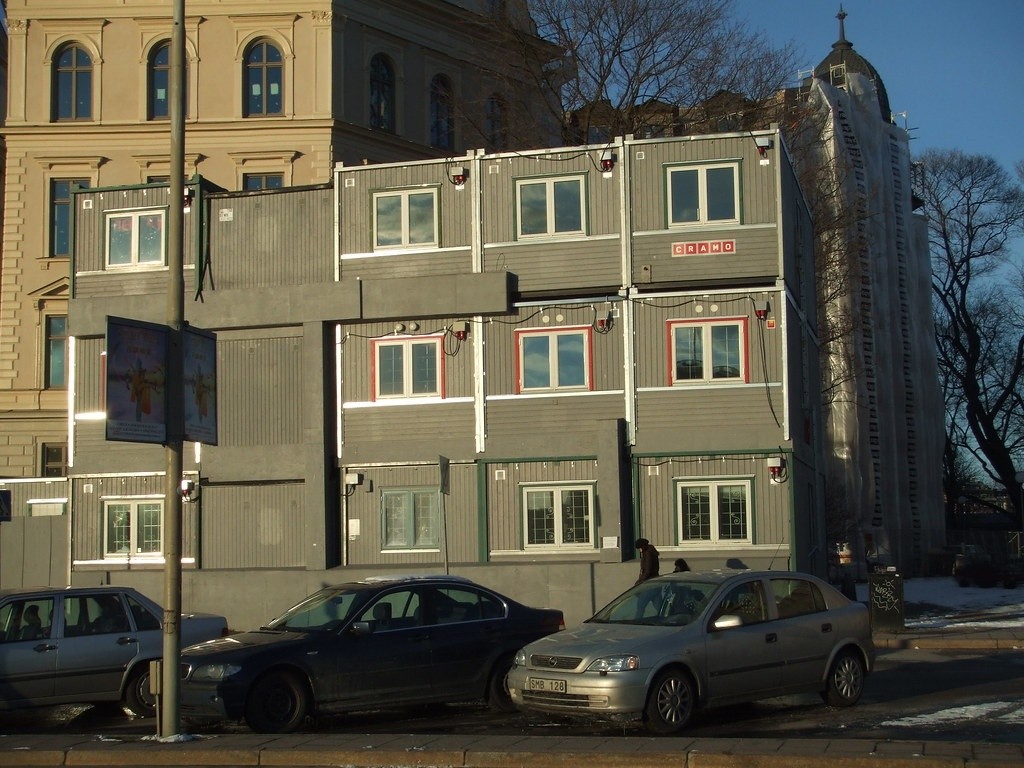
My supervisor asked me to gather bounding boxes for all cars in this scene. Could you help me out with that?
[0,584,230,718]
[508,568,876,737]
[179,574,567,735]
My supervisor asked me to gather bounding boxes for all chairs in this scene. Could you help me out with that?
[414,605,438,627]
[17,605,41,640]
[43,605,67,638]
[373,603,394,632]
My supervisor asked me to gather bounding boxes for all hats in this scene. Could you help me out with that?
[635,538,649,548]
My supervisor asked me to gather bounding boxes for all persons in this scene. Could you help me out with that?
[635,538,662,620]
